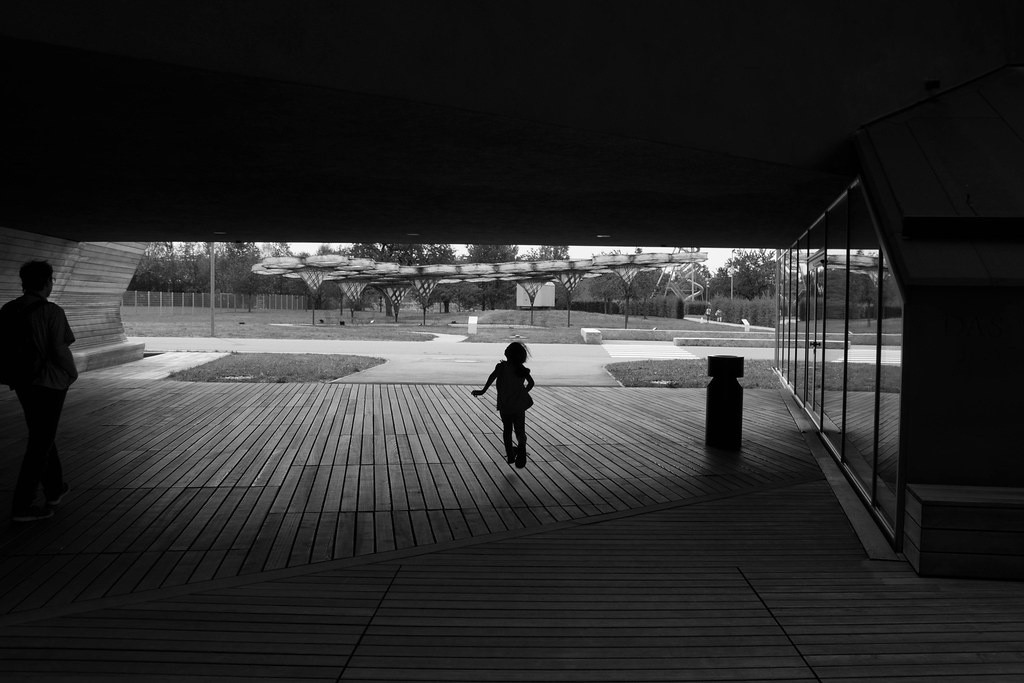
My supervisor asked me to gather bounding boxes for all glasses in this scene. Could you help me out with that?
[51,277,55,283]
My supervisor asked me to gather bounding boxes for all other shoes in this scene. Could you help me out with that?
[504,446,530,468]
[48,482,70,504]
[13,503,54,521]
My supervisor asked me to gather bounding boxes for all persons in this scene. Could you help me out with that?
[0,260,77,522]
[471,342,534,469]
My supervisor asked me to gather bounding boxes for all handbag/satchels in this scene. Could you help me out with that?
[509,390,533,410]
